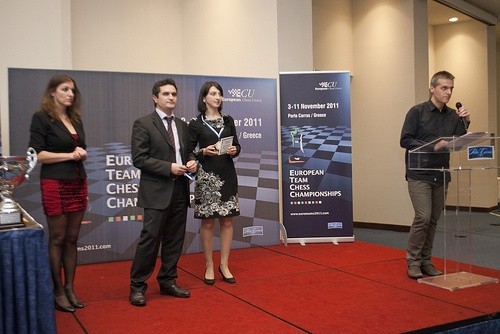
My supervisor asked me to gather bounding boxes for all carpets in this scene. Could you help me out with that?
[54,237,500,334]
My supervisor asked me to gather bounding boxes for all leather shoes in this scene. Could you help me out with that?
[160,284,190,298]
[129,290,146,306]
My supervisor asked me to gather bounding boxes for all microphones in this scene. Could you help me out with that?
[456,102,468,129]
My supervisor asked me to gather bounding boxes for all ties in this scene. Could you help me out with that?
[164,116,175,150]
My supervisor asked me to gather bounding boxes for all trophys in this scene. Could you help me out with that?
[0,147,38,225]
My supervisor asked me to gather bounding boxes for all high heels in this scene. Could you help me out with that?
[204,269,215,285]
[55,299,75,312]
[219,265,236,283]
[73,303,84,308]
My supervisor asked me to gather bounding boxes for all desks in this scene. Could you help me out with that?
[0,203,53,334]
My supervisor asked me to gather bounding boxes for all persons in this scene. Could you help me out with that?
[186,81,241,285]
[398,70,470,278]
[27,73,90,313]
[129,79,198,306]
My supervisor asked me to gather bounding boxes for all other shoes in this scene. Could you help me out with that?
[421,259,442,275]
[407,265,424,277]
